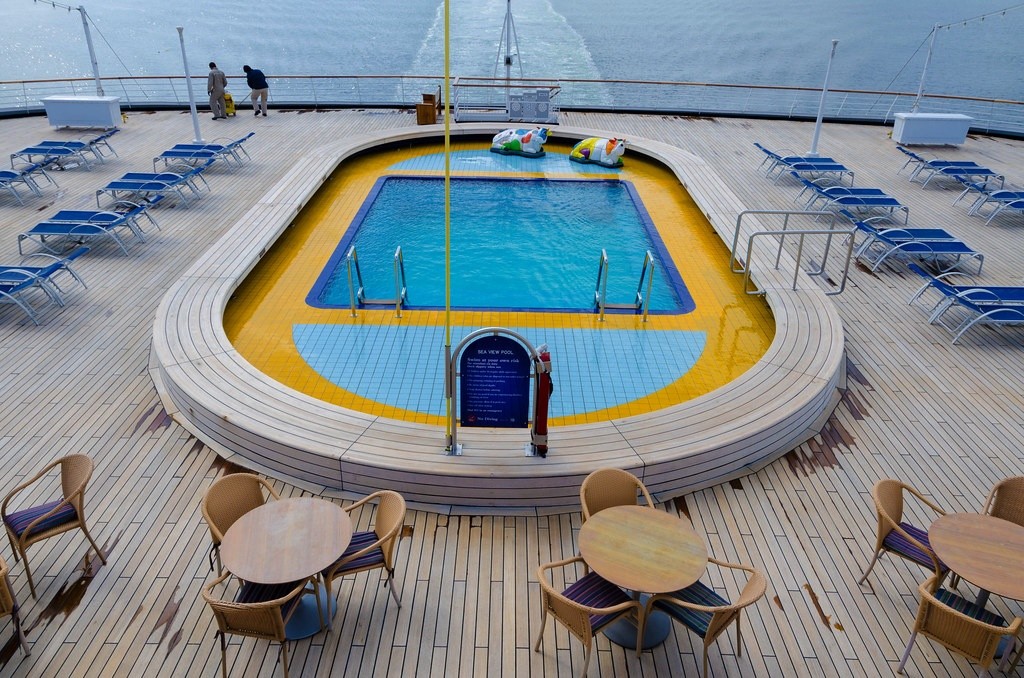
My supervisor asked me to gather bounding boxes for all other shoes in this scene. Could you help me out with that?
[220,117,226,119]
[262,113,267,116]
[212,116,221,120]
[254,110,260,115]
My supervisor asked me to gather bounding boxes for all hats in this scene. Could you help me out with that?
[244,65,251,72]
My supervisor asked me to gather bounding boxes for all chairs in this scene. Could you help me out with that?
[316,490,407,631]
[201,474,281,585]
[0,453,107,598]
[577,467,656,557]
[642,557,767,678]
[535,555,642,678]
[0,128,258,325]
[856,478,957,588]
[0,554,31,659]
[203,572,326,678]
[952,475,1024,589]
[897,576,1023,678]
[754,142,1024,345]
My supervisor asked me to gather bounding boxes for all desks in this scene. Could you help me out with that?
[220,496,353,640]
[577,505,707,653]
[40,95,121,132]
[891,112,974,147]
[927,512,1024,659]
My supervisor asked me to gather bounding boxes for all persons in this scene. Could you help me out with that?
[208,62,227,119]
[243,65,268,116]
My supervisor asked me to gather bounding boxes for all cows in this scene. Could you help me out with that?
[568,137,631,165]
[491,126,552,153]
